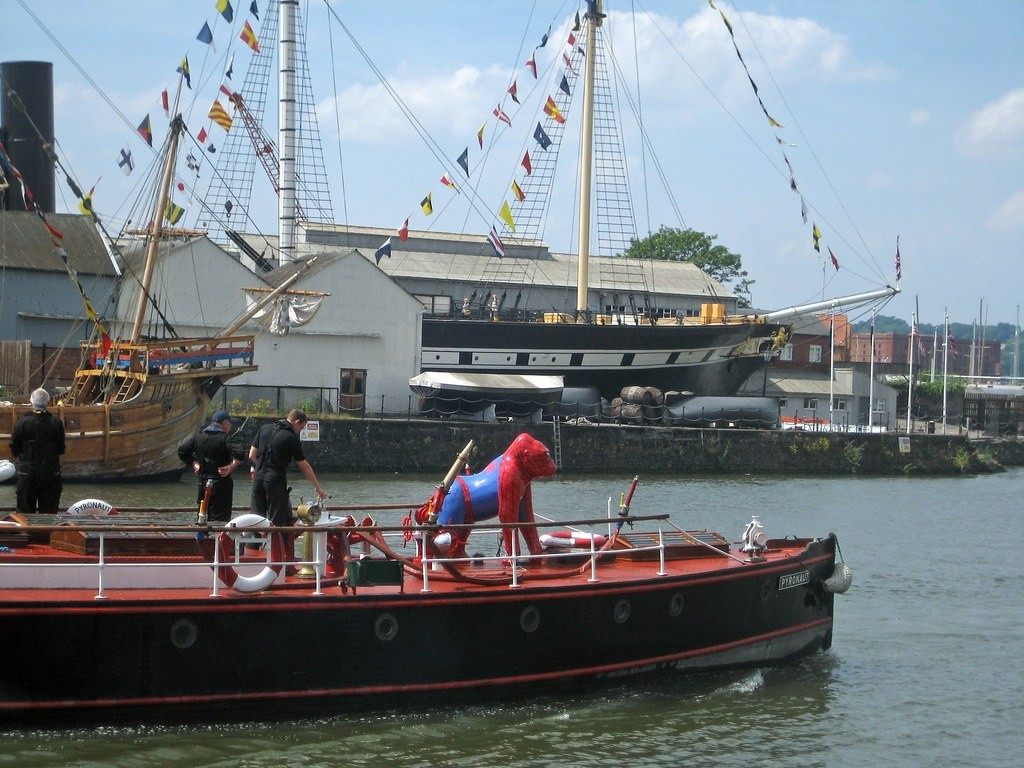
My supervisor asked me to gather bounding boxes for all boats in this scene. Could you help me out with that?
[0,440,852,733]
[409,370,564,416]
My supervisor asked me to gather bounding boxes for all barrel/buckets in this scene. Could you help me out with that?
[611,386,664,424]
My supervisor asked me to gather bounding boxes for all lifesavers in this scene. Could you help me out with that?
[90,350,97,369]
[540,531,606,547]
[215,513,285,593]
[144,350,166,372]
[66,498,121,534]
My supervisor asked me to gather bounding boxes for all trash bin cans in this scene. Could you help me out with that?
[928,421,935,433]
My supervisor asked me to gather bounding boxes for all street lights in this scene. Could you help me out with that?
[762,343,773,397]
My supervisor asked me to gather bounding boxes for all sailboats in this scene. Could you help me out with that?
[369,0,902,399]
[1,113,328,483]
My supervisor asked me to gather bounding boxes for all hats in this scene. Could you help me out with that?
[212,410,231,422]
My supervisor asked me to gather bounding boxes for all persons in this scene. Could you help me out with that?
[249,409,325,576]
[178,411,246,522]
[9,387,66,514]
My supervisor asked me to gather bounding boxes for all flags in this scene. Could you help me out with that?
[830,319,832,349]
[870,319,876,356]
[948,325,959,359]
[896,246,901,281]
[78,0,260,227]
[913,320,926,356]
[487,12,586,259]
[0,141,113,357]
[708,0,839,272]
[375,26,551,265]
